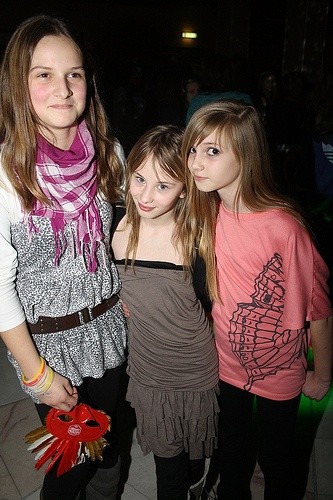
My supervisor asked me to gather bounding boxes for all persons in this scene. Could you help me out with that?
[174,51,333,201]
[111,59,151,144]
[171,101,333,500]
[0,15,139,500]
[107,125,220,500]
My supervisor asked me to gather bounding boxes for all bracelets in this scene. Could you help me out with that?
[21,357,54,396]
